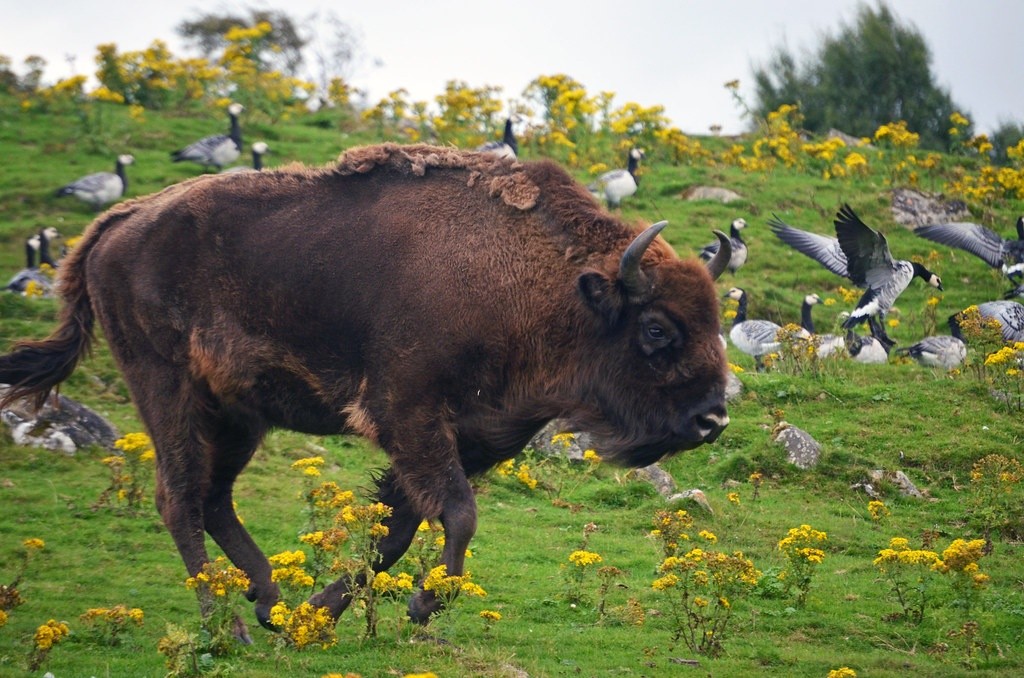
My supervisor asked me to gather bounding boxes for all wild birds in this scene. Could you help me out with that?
[0,101,1024,369]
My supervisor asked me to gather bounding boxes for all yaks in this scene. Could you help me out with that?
[0,143,733,643]
